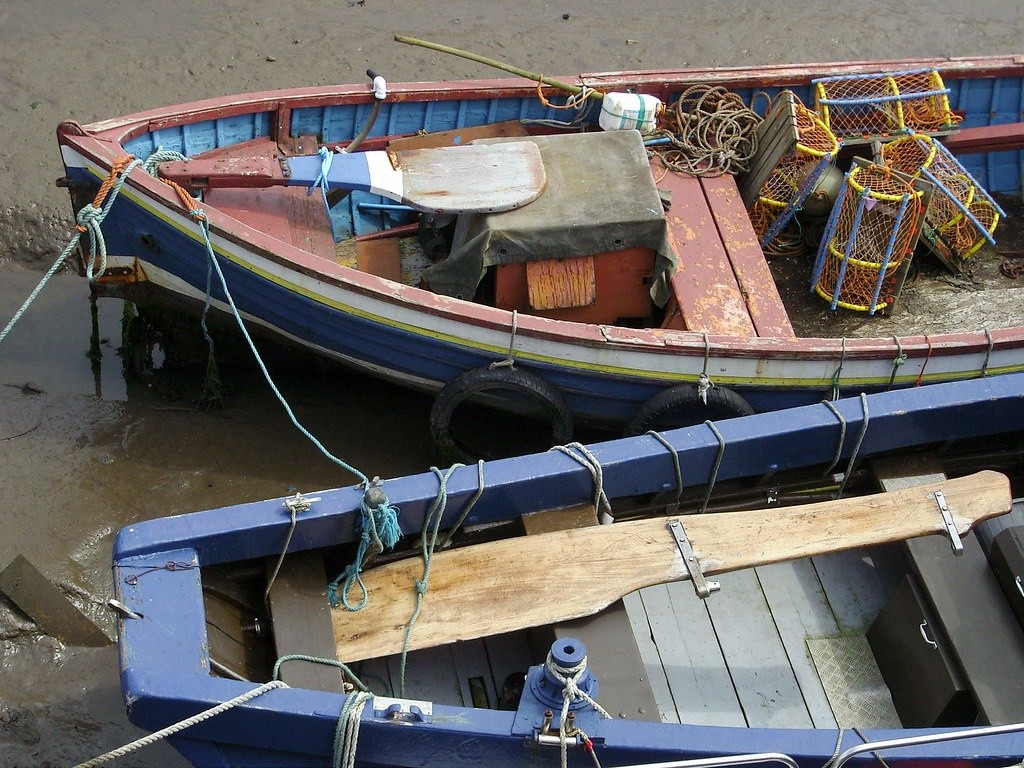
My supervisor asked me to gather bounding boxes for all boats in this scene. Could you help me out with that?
[112,367,1023,768]
[55,55,1024,430]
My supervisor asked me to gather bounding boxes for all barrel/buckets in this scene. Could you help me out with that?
[599,92,666,137]
